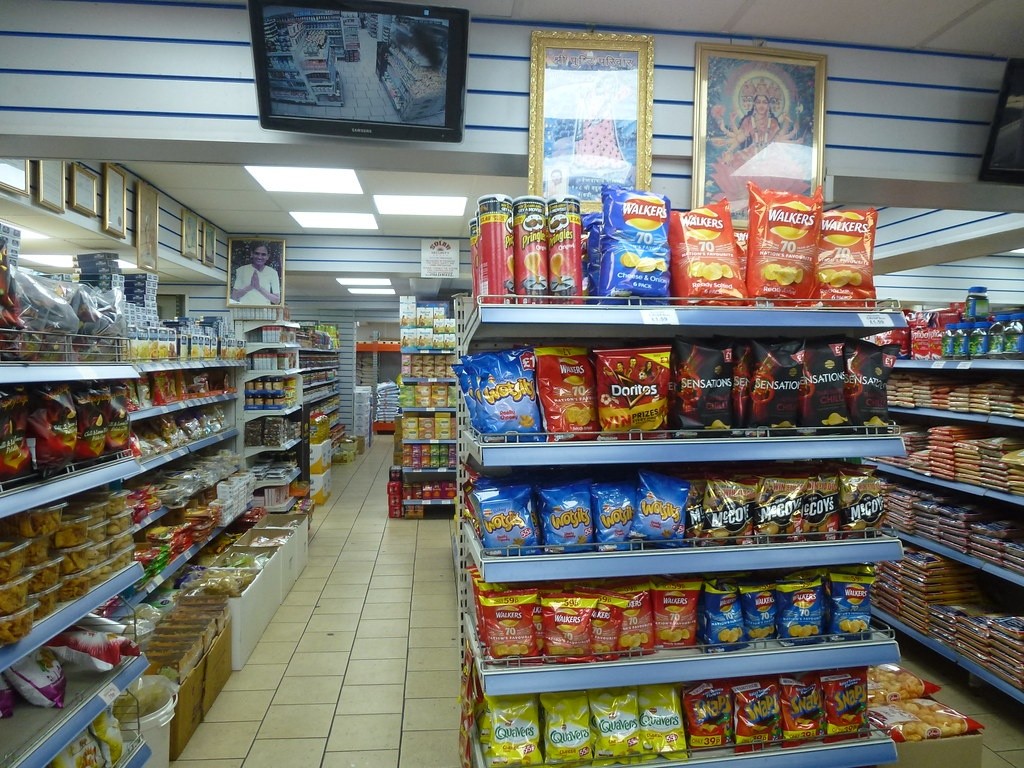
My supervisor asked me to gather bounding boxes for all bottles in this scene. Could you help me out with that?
[267,56,295,69]
[310,412,330,445]
[327,31,341,35]
[294,16,330,21]
[276,18,294,24]
[304,30,325,56]
[335,48,344,56]
[306,22,341,28]
[302,59,327,69]
[307,73,330,83]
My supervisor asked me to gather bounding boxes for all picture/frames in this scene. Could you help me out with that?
[135,180,160,275]
[102,163,127,240]
[70,163,98,218]
[36,159,66,214]
[977,58,1024,186]
[202,221,217,268]
[181,208,200,259]
[226,237,286,309]
[689,41,827,229]
[529,30,656,214]
[0,159,31,198]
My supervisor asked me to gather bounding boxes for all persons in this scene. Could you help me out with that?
[231,244,280,306]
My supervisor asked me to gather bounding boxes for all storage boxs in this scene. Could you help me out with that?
[0,224,247,363]
[869,695,984,768]
[310,436,365,506]
[0,489,135,649]
[137,458,309,763]
[388,295,457,521]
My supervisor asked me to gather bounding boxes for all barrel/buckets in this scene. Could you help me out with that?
[115,677,178,768]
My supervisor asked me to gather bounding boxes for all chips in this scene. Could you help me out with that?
[705,411,889,430]
[495,618,867,656]
[622,250,861,287]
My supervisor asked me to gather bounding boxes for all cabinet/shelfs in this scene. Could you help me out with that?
[300,347,341,429]
[356,330,401,430]
[0,328,154,768]
[861,359,1024,706]
[451,288,901,768]
[400,347,457,506]
[133,360,252,594]
[235,320,302,512]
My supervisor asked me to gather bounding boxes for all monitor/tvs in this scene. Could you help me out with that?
[247,0,471,143]
[977,58,1024,185]
